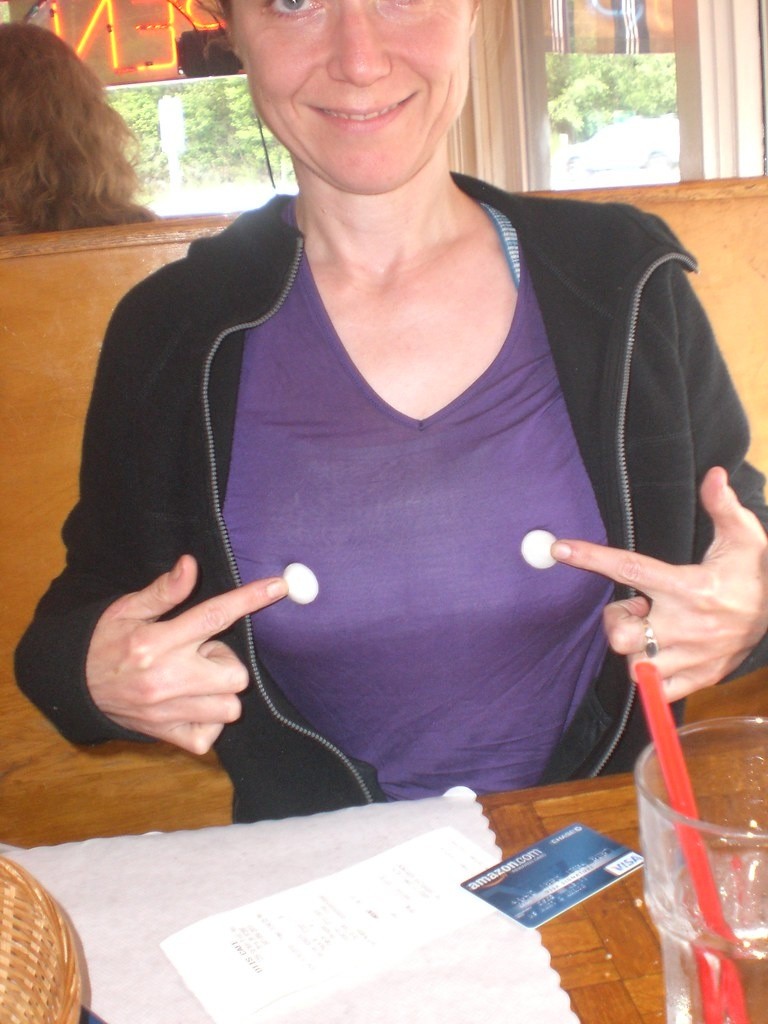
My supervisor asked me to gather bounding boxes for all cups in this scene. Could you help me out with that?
[633,718,768,1024]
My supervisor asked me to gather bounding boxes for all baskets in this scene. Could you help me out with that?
[0,856,82,1024]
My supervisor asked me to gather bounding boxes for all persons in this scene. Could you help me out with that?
[12,1,768,828]
[0,21,160,237]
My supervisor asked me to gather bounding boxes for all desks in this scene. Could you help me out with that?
[0,745,768,1024]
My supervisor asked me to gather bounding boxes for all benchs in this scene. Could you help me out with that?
[0,177,768,849]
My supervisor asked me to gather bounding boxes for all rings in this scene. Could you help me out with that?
[643,619,661,661]
